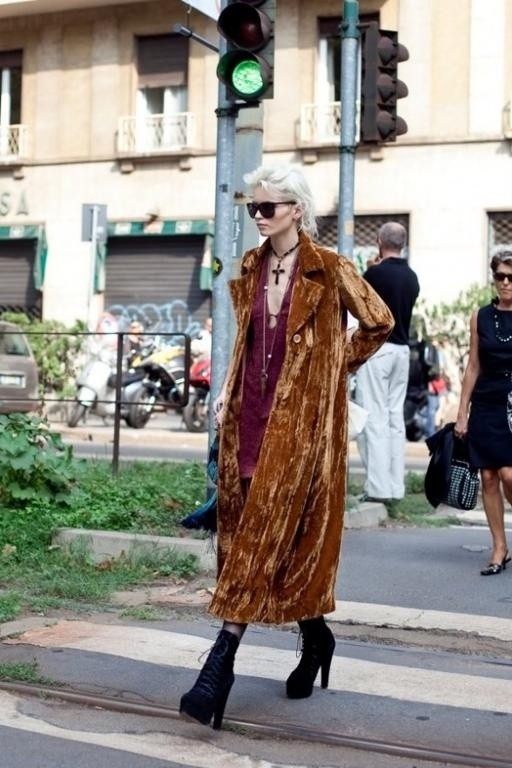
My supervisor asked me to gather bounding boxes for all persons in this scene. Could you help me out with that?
[418,340,450,442]
[196,316,214,340]
[452,247,512,579]
[177,159,400,730]
[353,221,420,504]
[125,318,157,351]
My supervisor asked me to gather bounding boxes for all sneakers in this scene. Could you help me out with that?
[358,493,400,507]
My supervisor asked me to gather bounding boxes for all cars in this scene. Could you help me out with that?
[1,317,41,419]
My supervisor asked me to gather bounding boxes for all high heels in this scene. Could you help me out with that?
[480,549,512,576]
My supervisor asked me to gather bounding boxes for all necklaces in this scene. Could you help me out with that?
[493,302,511,344]
[260,281,285,397]
[267,241,299,284]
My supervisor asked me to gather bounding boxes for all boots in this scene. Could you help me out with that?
[286,615,336,700]
[179,627,241,731]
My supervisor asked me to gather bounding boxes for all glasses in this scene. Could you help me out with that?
[246,199,297,219]
[494,272,512,283]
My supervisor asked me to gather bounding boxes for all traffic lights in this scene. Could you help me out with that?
[211,1,279,105]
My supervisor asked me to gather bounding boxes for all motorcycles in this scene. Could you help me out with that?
[63,314,215,433]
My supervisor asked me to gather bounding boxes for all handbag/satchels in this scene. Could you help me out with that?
[440,435,480,511]
